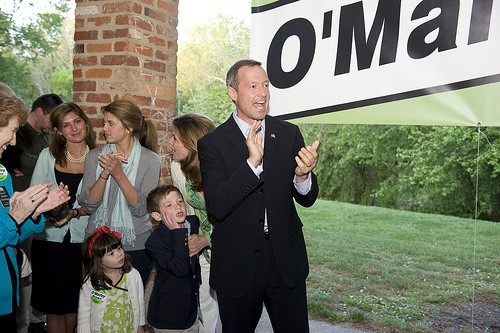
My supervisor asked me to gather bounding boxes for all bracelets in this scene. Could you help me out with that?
[100,174,108,180]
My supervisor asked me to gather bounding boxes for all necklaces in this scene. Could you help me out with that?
[64,143,90,163]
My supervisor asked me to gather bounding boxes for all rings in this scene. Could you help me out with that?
[30,199,35,202]
[188,249,192,253]
[306,164,311,167]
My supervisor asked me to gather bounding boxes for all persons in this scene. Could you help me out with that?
[197,60,320,333]
[0,81,217,333]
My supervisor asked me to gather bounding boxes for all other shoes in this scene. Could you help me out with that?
[27,321,48,333]
